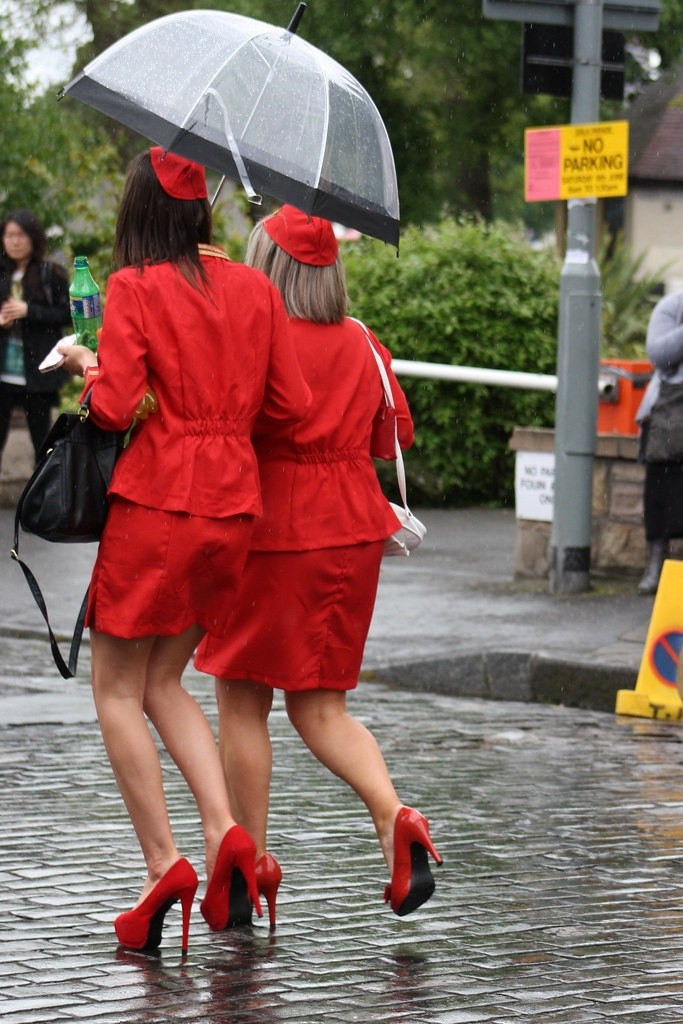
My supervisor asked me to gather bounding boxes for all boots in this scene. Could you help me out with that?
[638,541,670,596]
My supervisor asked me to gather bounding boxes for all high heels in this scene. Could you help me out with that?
[384,806,443,917]
[256,853,282,927]
[114,858,199,951]
[199,826,264,932]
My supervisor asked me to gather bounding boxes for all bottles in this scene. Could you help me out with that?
[96,326,159,420]
[68,257,107,363]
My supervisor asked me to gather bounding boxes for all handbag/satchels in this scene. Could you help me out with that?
[382,502,428,558]
[10,385,138,680]
[640,378,683,463]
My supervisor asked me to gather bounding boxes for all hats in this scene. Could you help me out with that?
[263,203,339,266]
[149,144,208,200]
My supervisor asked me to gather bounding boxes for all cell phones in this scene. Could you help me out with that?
[39,332,79,374]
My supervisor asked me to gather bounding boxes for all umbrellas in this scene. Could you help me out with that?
[56,1,401,260]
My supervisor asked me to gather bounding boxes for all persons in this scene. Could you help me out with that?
[0,207,72,484]
[57,146,264,954]
[191,205,442,934]
[632,286,683,596]
